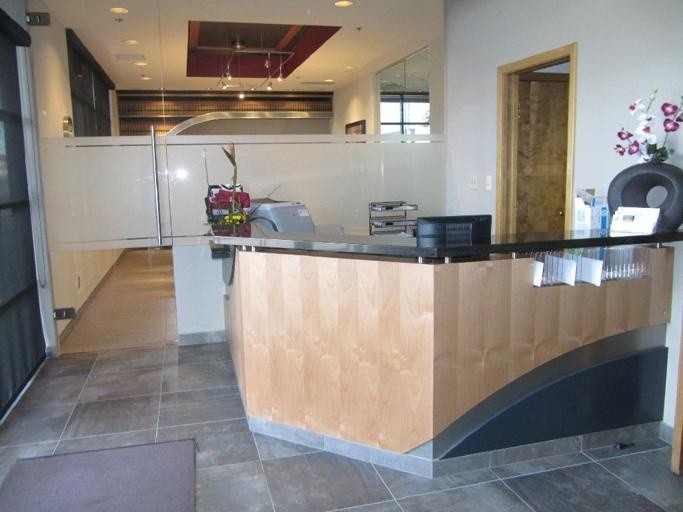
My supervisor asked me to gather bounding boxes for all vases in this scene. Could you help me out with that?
[607,161,683,235]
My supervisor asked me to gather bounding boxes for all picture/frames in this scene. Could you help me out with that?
[344,119,365,144]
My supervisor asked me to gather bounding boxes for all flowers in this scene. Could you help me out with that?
[198,143,282,235]
[613,87,682,164]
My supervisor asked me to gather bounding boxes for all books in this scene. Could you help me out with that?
[371,200,417,211]
[610,206,661,236]
[374,230,413,238]
[371,215,417,228]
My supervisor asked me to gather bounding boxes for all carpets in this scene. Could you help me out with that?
[0,439,195,512]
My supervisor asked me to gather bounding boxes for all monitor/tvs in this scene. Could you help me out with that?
[417,215,492,244]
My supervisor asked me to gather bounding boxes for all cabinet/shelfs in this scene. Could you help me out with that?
[368,201,417,236]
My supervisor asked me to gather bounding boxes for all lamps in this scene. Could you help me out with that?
[267,52,272,90]
[226,36,231,79]
[277,28,283,82]
[221,61,227,89]
